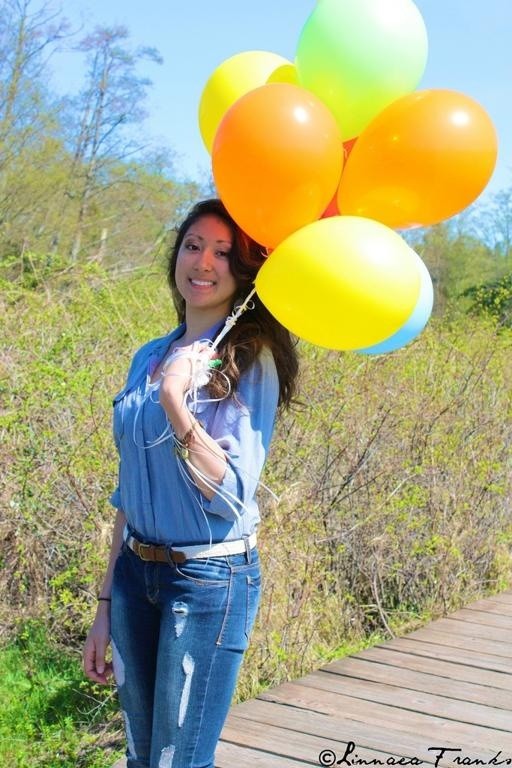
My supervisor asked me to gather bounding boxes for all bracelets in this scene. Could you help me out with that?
[97,597,111,602]
[174,423,198,459]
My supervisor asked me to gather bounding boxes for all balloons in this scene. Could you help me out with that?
[253,215,421,351]
[337,89,498,229]
[296,0,428,139]
[198,51,295,154]
[213,83,343,250]
[321,139,358,218]
[357,254,434,355]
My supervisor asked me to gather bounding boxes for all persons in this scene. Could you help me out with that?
[80,198,300,768]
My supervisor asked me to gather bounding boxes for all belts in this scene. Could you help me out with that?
[122,522,258,563]
